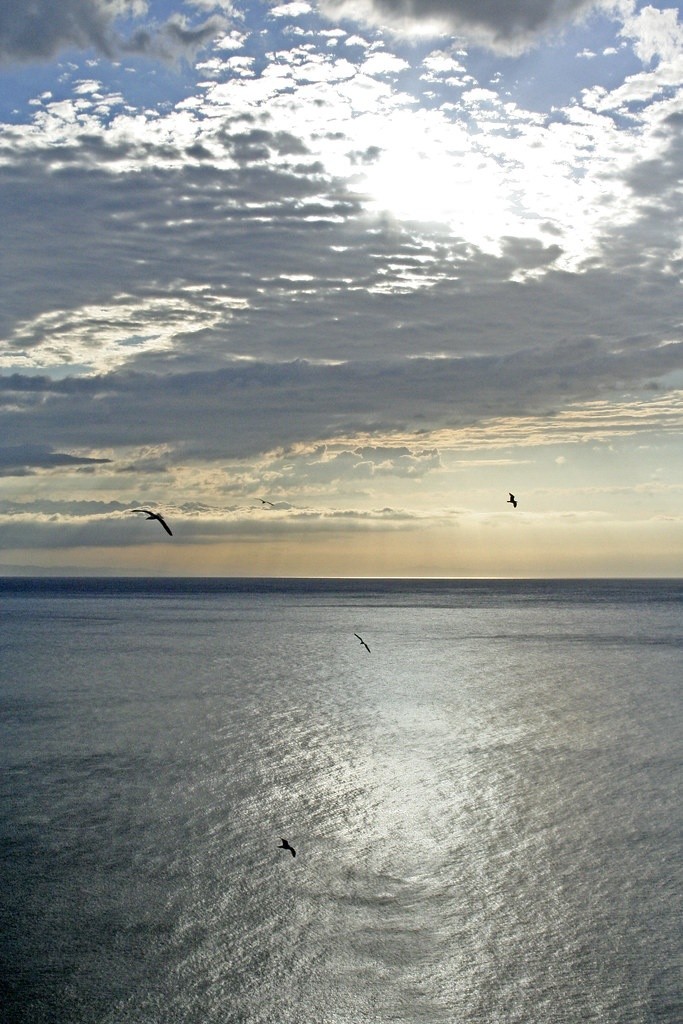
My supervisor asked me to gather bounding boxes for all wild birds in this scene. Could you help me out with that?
[507,493,518,508]
[253,498,275,507]
[277,836,297,858]
[352,632,371,654]
[129,509,174,537]
